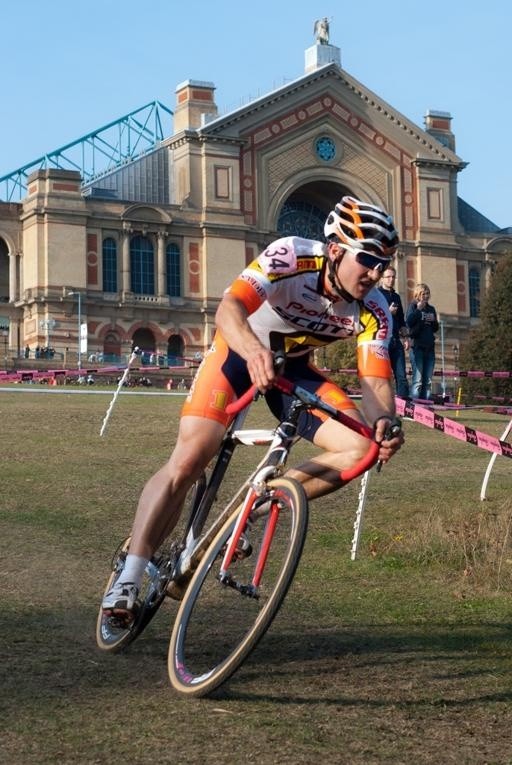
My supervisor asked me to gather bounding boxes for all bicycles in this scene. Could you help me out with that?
[95,373,403,700]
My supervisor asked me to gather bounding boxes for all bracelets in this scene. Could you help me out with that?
[375,413,393,430]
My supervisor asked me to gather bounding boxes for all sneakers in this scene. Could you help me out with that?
[219,518,252,562]
[102,583,138,627]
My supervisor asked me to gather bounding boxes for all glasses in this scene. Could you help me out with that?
[337,243,393,273]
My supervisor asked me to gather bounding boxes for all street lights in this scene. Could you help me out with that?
[453,344,459,403]
[67,291,82,382]
[2,329,10,374]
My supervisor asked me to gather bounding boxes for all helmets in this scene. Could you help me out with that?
[324,195,400,255]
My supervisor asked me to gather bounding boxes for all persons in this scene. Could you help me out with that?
[158,352,164,366]
[39,372,189,391]
[100,193,408,632]
[23,342,56,360]
[149,353,157,366]
[405,283,441,404]
[314,16,335,44]
[377,265,412,403]
